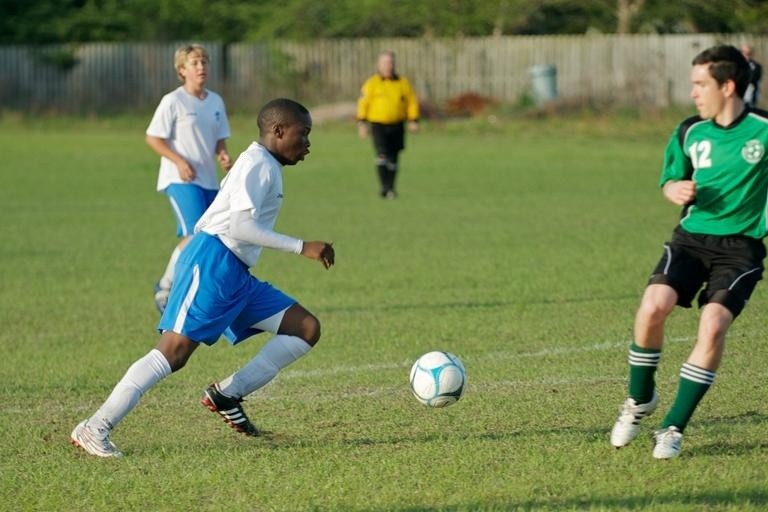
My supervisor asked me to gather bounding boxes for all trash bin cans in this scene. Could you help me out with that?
[528,63,557,102]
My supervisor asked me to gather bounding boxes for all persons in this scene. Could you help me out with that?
[144,42,234,317]
[70,99,335,457]
[740,45,763,110]
[610,44,768,462]
[355,50,421,201]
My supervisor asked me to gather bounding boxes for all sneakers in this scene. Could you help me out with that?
[609,389,658,448]
[68,417,124,459]
[151,280,172,315]
[651,424,685,461]
[199,381,259,439]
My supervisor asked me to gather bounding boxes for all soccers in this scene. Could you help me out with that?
[409,351,467,410]
